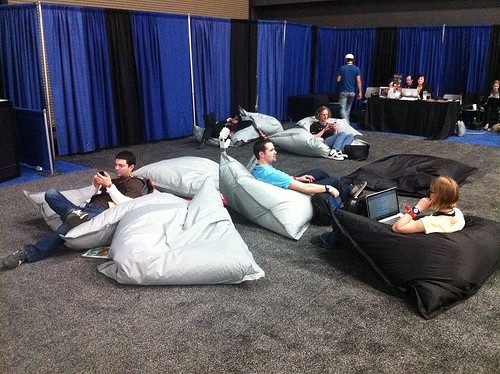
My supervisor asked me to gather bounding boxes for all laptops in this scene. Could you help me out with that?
[365,187,404,225]
[401,88,418,98]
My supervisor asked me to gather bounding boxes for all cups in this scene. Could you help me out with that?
[423,91,427,100]
[473,104,477,110]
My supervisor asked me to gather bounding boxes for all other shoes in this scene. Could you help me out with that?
[197,139,207,150]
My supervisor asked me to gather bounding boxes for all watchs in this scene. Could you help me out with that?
[412,206,421,215]
[325,185,329,193]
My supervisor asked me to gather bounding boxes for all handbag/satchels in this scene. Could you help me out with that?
[312,191,333,226]
[457,120,465,137]
[345,144,369,160]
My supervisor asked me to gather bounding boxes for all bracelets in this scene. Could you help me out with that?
[405,205,416,219]
[106,183,113,191]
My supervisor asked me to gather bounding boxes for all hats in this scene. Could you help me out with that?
[345,54,354,59]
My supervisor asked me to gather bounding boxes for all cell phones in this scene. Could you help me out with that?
[98,170,104,176]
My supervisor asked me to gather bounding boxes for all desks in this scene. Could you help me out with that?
[288,93,329,122]
[367,95,460,139]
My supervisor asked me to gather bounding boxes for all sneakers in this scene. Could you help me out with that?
[336,150,348,158]
[2,248,27,269]
[350,180,368,199]
[328,150,344,160]
[66,208,88,227]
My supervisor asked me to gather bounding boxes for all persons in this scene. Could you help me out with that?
[403,75,414,88]
[197,112,253,150]
[2,151,149,269]
[391,176,465,235]
[478,80,500,130]
[411,73,432,99]
[337,54,362,125]
[251,138,368,202]
[310,106,354,161]
